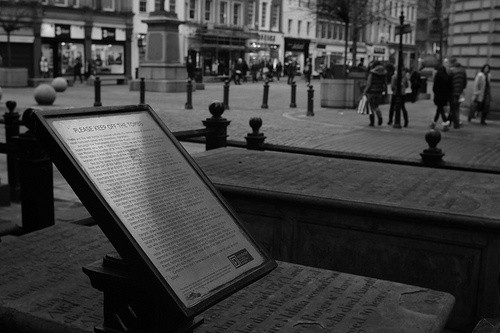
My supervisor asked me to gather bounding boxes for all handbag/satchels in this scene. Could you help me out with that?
[356,95,373,115]
[379,94,390,104]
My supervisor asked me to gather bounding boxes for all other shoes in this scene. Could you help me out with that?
[441,125,449,133]
[404,120,408,128]
[429,123,435,129]
[387,120,392,125]
[468,115,471,122]
[368,123,374,127]
[480,120,487,125]
[378,117,382,126]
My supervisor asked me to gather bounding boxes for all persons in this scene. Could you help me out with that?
[39,56,49,78]
[431,65,450,131]
[222,56,313,84]
[468,64,492,125]
[364,61,388,126]
[448,58,467,129]
[386,66,421,128]
[70,54,102,84]
[185,54,195,81]
